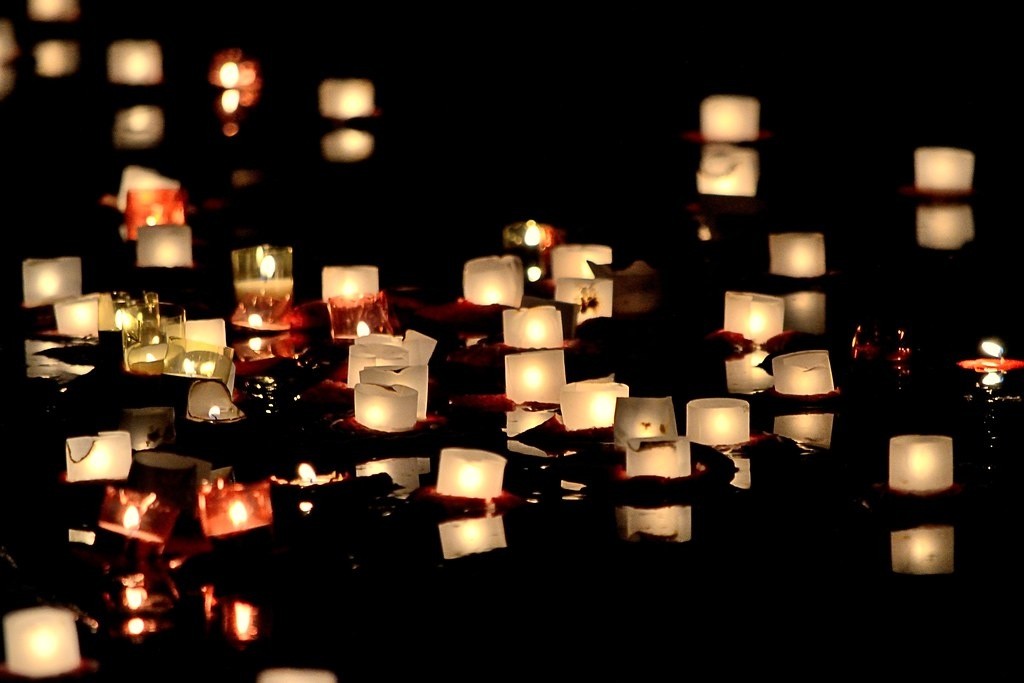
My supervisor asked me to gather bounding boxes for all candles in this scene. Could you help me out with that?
[93,256,357,579]
[956,337,1024,372]
